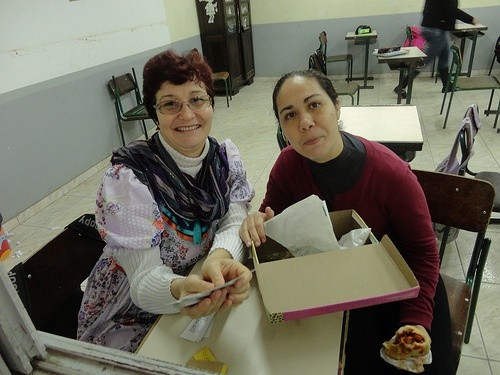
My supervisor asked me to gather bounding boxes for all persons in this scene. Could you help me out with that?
[78,49,256,353]
[239,69,454,375]
[394,0,478,99]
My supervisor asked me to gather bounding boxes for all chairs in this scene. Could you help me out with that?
[108,68,153,147]
[8,214,107,341]
[277,25,500,375]
[192,48,232,107]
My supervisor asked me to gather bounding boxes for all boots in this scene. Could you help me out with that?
[439,66,457,93]
[393,67,420,98]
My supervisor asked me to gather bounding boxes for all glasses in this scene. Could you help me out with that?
[153,94,212,115]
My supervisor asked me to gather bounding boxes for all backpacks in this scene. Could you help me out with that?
[429,104,480,244]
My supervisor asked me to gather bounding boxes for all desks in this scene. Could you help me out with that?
[135,252,342,375]
[345,30,378,89]
[337,107,423,157]
[375,46,428,104]
[436,23,488,77]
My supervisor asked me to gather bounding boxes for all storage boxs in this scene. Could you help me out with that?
[250,209,420,325]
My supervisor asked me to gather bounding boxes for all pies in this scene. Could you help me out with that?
[383,325,430,360]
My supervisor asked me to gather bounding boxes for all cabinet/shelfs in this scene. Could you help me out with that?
[195,0,255,95]
